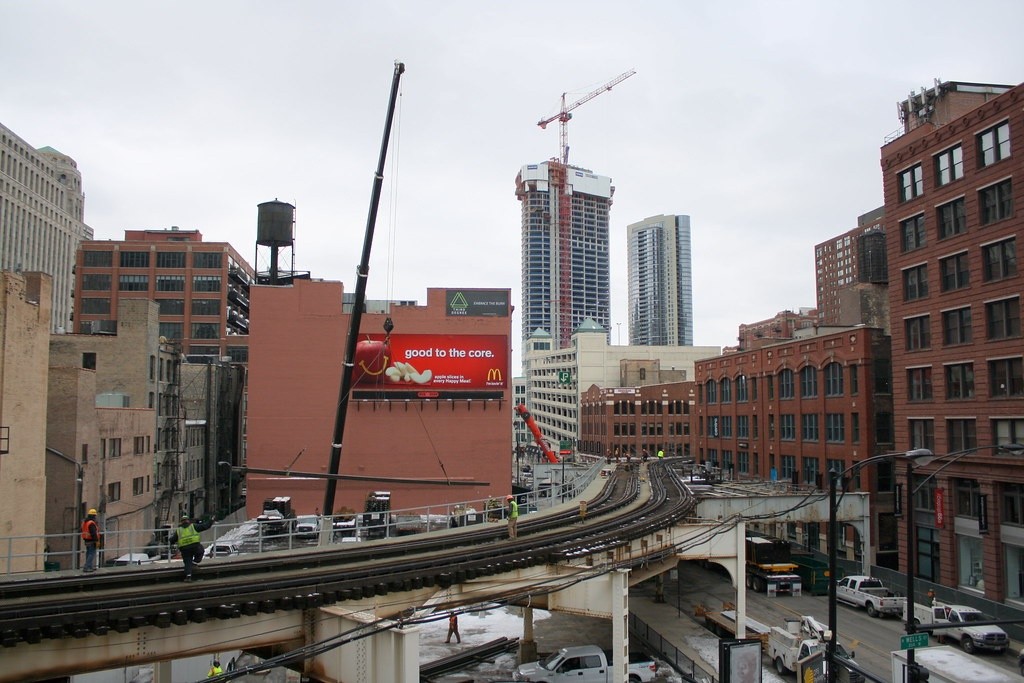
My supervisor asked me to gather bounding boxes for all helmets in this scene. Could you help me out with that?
[214,659,219,663]
[506,495,514,500]
[88,509,98,516]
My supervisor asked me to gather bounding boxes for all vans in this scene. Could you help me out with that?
[113,552,148,566]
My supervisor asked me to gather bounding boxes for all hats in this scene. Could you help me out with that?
[181,516,190,521]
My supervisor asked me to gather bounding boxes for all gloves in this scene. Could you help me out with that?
[96,541,100,548]
[507,517,511,521]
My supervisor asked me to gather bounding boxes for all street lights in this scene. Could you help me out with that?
[906,443,1024,683]
[828,449,934,683]
[218,461,232,515]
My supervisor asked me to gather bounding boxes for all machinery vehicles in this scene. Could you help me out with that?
[761,616,865,682]
[318,62,405,547]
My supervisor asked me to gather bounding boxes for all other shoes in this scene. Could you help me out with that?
[83,569,93,572]
[445,641,450,643]
[457,642,460,643]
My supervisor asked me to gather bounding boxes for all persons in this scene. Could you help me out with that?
[207,658,224,678]
[606,447,664,464]
[504,494,519,541]
[82,509,101,573]
[168,515,216,583]
[444,610,462,643]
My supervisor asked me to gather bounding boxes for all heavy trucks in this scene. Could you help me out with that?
[746,537,802,598]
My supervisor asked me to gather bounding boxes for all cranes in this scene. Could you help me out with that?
[537,69,637,165]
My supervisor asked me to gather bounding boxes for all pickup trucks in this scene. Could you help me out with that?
[204,544,239,559]
[836,576,907,617]
[903,598,1010,654]
[513,644,656,683]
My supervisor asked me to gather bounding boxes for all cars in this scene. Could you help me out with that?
[295,515,318,539]
